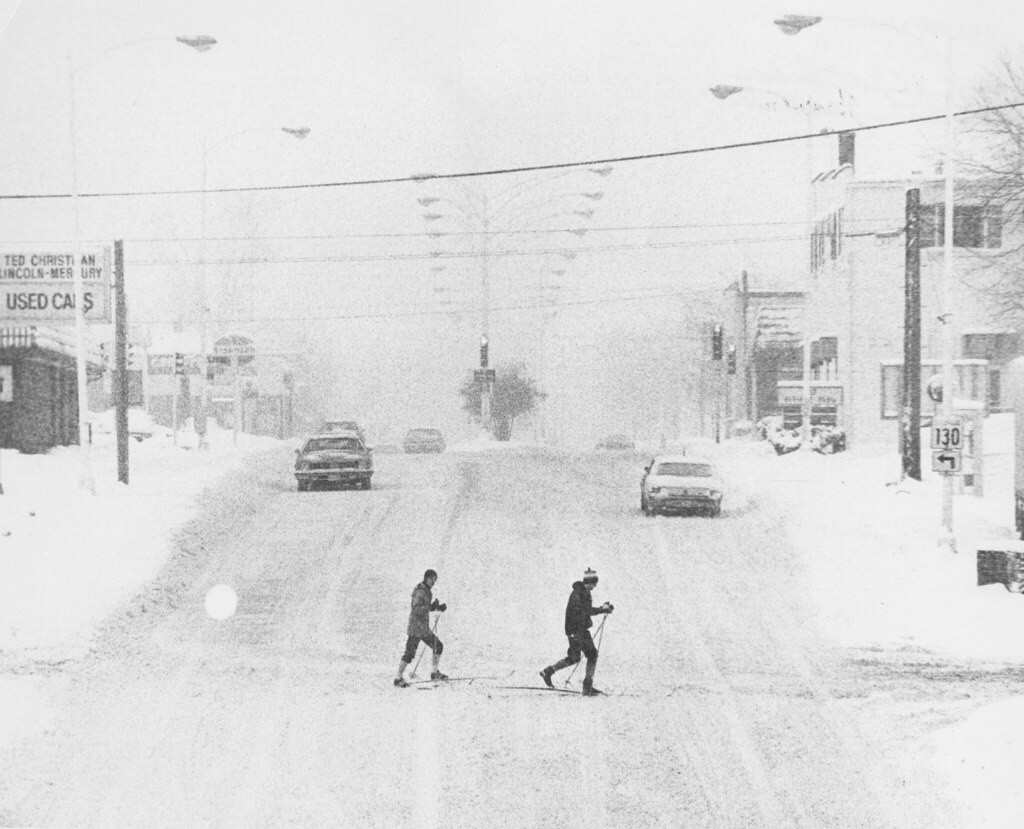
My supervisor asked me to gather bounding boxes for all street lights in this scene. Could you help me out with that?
[75,33,219,481]
[399,160,615,443]
[775,12,954,417]
[198,121,313,460]
[709,78,804,115]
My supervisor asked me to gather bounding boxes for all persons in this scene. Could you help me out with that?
[539,567,614,696]
[394,569,447,688]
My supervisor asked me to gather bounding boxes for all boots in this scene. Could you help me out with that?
[583,679,603,696]
[540,665,554,688]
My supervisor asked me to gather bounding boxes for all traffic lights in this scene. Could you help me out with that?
[727,347,737,374]
[713,323,725,361]
[480,333,491,368]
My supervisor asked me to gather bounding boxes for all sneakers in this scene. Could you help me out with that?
[431,670,448,680]
[394,677,408,688]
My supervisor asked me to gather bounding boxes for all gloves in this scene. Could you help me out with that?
[438,603,446,612]
[600,602,614,613]
[430,600,439,610]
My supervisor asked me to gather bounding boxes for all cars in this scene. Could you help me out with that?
[401,424,448,451]
[292,428,379,494]
[638,457,726,522]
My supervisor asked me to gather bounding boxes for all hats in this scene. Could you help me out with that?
[583,567,598,582]
